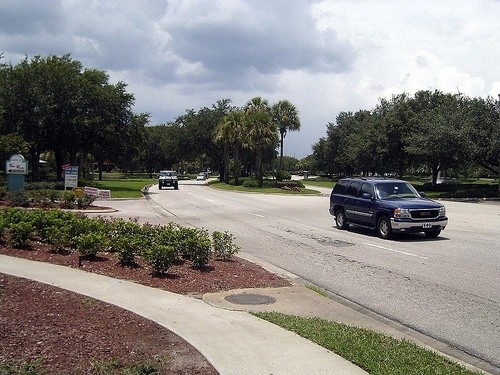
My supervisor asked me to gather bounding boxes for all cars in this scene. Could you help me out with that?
[197,172,207,181]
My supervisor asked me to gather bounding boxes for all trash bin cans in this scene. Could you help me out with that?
[303,170,309,181]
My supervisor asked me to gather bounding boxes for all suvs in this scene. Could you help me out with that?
[329,174,449,240]
[157,170,179,190]
